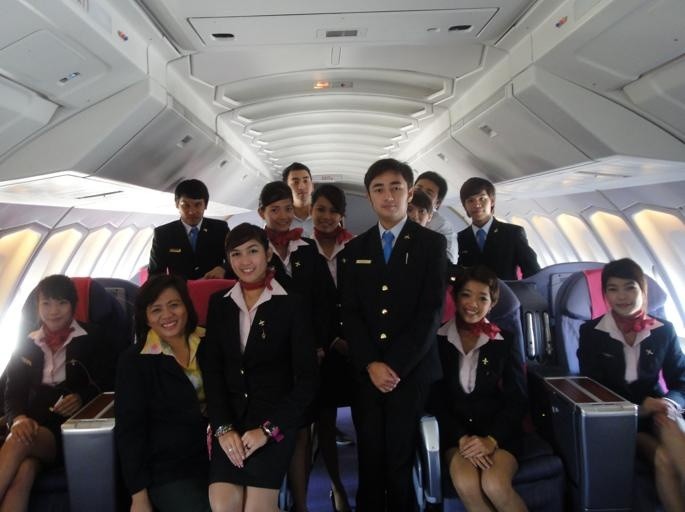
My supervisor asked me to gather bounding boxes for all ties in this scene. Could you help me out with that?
[476,229,487,251]
[383,231,394,265]
[190,227,199,250]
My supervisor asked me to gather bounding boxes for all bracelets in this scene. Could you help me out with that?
[488,435,496,443]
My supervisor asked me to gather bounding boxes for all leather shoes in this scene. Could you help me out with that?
[330,488,351,511]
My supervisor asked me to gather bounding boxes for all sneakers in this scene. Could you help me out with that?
[335,433,353,446]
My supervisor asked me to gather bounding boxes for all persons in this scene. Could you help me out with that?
[437,267,530,511]
[339,158,446,511]
[1,275,110,511]
[114,161,356,511]
[407,173,543,282]
[576,258,685,511]
